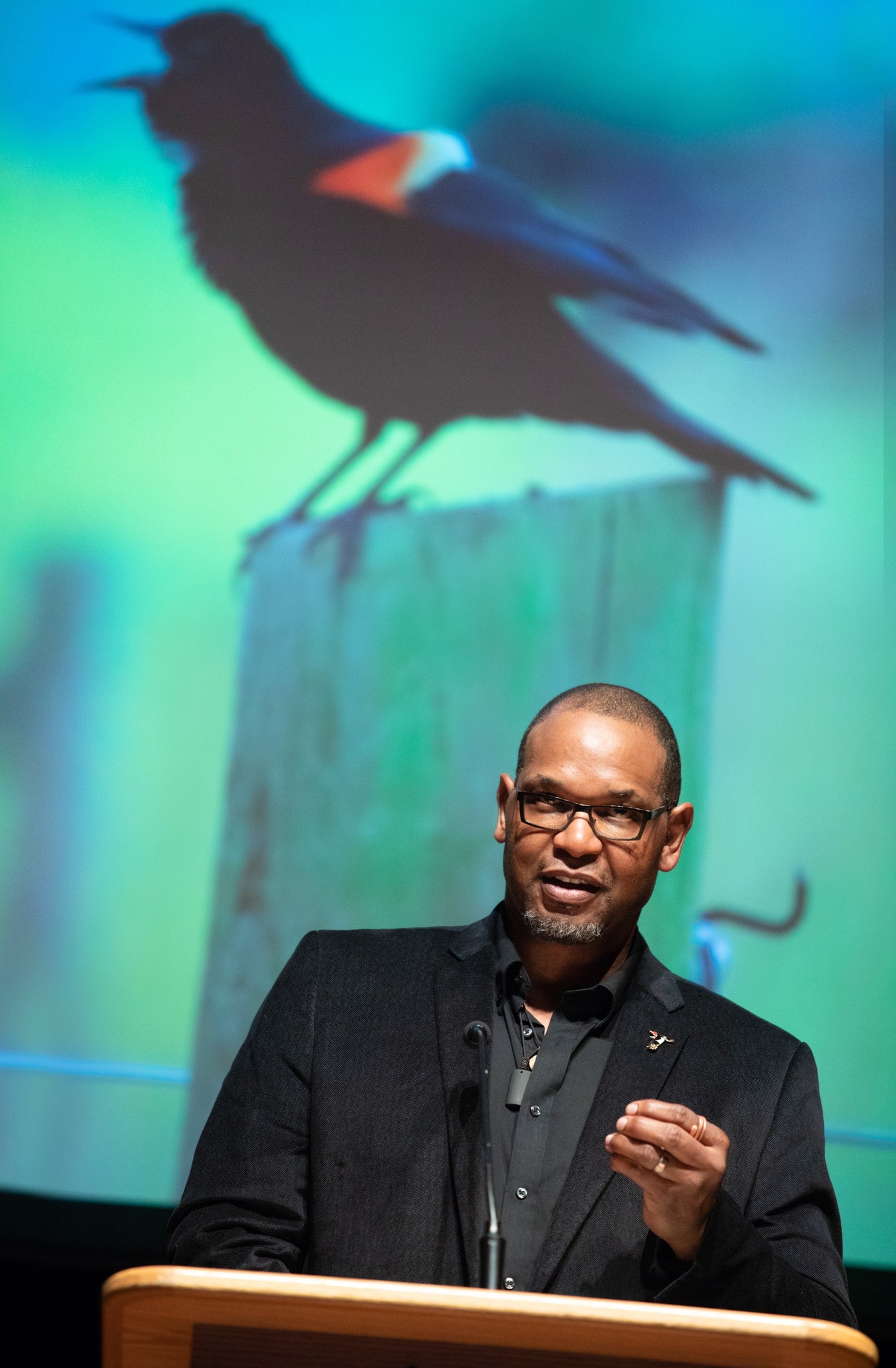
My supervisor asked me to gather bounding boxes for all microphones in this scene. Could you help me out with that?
[462,1019,507,1290]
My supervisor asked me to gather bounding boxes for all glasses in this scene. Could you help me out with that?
[510,782,670,847]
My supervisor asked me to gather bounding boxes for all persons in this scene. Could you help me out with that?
[168,683,860,1329]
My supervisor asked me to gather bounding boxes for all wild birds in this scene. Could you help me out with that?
[75,10,821,590]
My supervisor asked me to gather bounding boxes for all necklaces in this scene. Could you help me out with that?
[505,998,542,1106]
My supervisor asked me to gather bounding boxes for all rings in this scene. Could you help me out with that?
[690,1115,707,1141]
[652,1154,670,1174]
